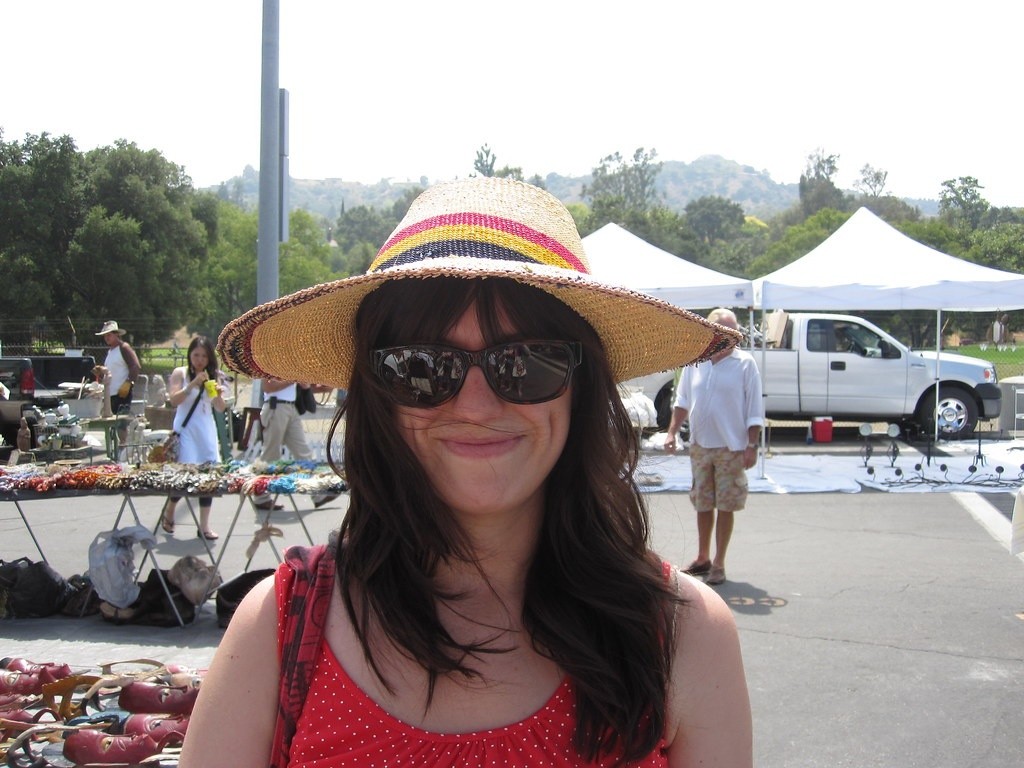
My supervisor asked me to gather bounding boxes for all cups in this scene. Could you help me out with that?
[204,379,217,400]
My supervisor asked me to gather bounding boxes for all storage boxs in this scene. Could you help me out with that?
[811,416,833,442]
[32,418,91,448]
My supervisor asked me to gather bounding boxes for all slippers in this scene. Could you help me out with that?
[198,527,220,540]
[163,510,176,533]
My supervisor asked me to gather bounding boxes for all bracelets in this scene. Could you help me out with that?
[747,442,758,451]
[129,378,135,386]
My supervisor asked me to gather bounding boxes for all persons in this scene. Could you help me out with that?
[249,372,354,511]
[162,335,227,541]
[657,303,763,589]
[177,169,760,768]
[90,321,141,462]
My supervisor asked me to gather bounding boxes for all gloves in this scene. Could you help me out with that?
[118,382,130,398]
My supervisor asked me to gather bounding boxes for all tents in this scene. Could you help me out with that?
[577,220,758,361]
[754,205,1024,442]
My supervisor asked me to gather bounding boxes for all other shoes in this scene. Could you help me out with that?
[678,558,712,577]
[255,501,284,510]
[704,566,727,584]
[314,492,341,508]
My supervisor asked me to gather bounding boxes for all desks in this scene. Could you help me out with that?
[0,465,352,627]
[30,445,93,466]
[144,402,177,431]
[81,414,128,462]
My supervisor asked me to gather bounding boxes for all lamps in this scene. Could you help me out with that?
[857,423,1024,489]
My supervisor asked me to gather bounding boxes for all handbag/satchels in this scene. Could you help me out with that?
[147,433,181,466]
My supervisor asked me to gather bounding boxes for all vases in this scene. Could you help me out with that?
[100,378,114,417]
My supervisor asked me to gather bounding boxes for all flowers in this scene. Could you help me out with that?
[91,366,113,384]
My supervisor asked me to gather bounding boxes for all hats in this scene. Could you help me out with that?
[217,175,744,388]
[95,320,127,336]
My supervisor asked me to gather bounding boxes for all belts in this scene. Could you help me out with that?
[264,399,298,405]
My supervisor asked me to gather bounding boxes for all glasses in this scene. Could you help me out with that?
[365,331,588,404]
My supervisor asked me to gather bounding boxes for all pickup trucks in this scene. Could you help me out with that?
[616,311,1002,441]
[1,356,102,450]
